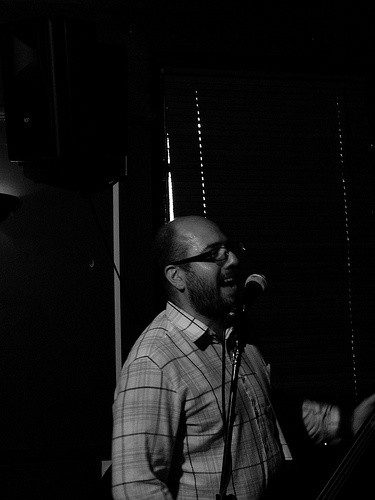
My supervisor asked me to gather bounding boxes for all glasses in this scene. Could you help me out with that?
[171,241,247,265]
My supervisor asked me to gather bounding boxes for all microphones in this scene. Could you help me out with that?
[228,273,267,319]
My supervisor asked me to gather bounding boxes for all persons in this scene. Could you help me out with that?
[112,215,375,500]
